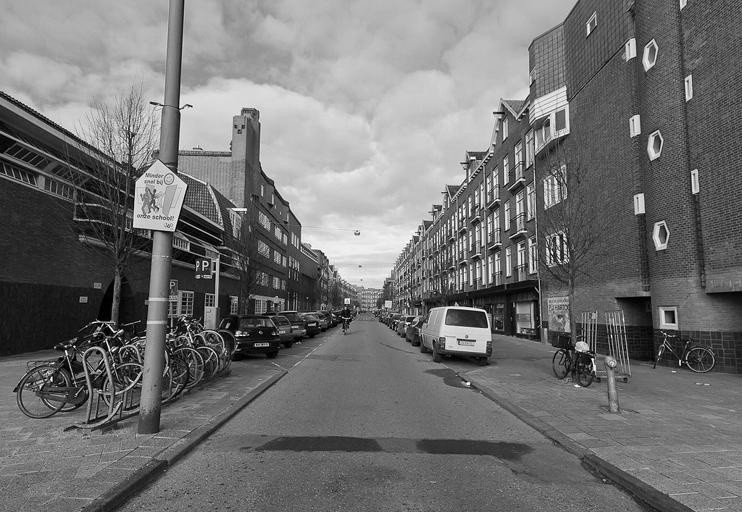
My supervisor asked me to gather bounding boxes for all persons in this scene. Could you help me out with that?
[341,306,352,331]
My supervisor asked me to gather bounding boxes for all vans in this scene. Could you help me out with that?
[419,307,493,366]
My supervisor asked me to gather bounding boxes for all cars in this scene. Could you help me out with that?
[375,304,426,346]
[217,307,357,361]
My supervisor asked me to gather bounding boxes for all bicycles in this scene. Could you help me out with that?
[551,333,596,387]
[339,316,351,335]
[653,328,716,373]
[12,313,239,419]
